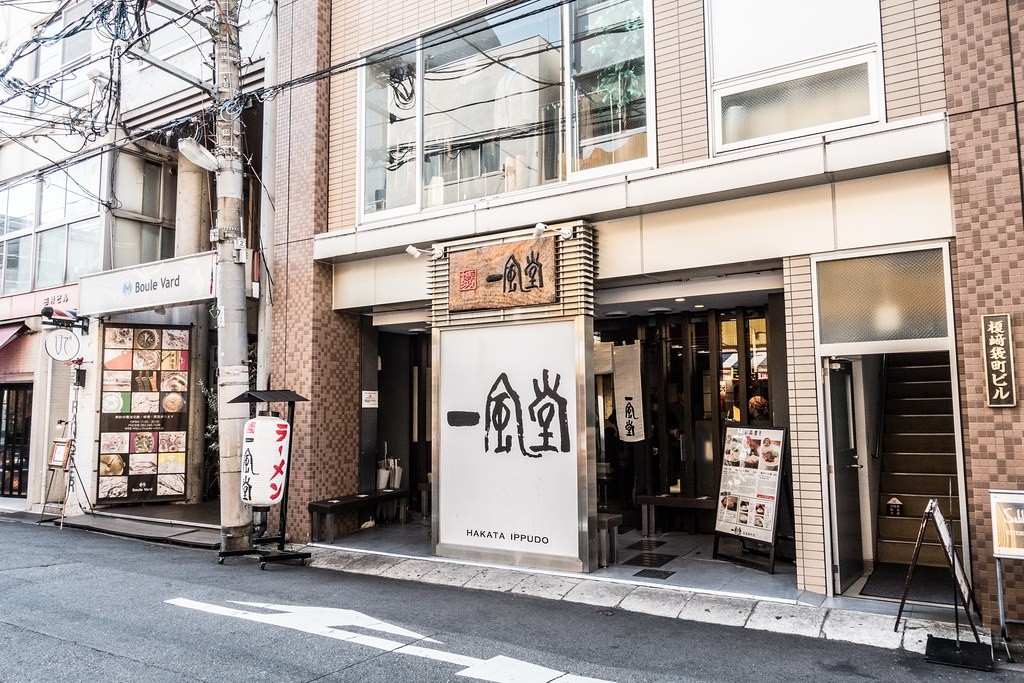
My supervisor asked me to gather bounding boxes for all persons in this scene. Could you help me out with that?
[749,395,771,427]
[605,381,690,512]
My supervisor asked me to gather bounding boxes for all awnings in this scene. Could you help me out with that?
[0,321,27,349]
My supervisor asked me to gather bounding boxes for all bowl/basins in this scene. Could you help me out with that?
[761,445,780,470]
[724,441,747,462]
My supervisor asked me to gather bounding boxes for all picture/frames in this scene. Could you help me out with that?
[47,440,71,467]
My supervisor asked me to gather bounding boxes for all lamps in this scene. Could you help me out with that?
[406,244,444,259]
[533,222,572,240]
[56,420,68,429]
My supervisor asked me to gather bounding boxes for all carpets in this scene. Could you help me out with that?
[859,562,964,606]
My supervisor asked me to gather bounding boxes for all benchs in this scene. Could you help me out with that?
[597,513,623,568]
[308,488,409,544]
[636,495,717,538]
